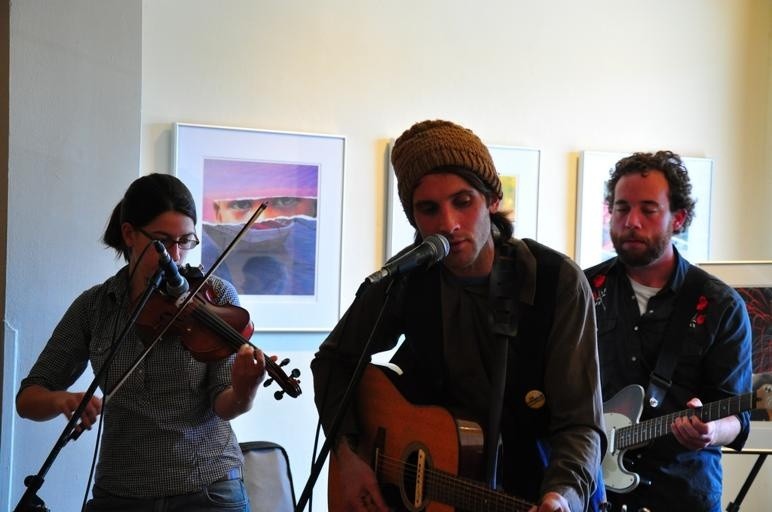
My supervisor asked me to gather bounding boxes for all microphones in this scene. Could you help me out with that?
[154,240,189,298]
[365,234,450,289]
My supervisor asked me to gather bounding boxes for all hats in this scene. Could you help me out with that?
[393,120,502,228]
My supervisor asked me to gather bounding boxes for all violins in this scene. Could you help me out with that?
[128,266,302,400]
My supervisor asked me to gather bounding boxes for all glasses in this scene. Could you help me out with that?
[135,227,199,250]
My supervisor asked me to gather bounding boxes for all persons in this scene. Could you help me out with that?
[16,172,278,512]
[582,150,752,511]
[310,119,607,511]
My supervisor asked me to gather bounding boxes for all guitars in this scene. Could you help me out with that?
[602,383,772,494]
[327,363,652,512]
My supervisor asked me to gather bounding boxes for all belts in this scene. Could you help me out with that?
[213,467,243,482]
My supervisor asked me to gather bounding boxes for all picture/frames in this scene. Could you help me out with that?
[573,149,713,273]
[170,120,346,335]
[382,140,540,262]
